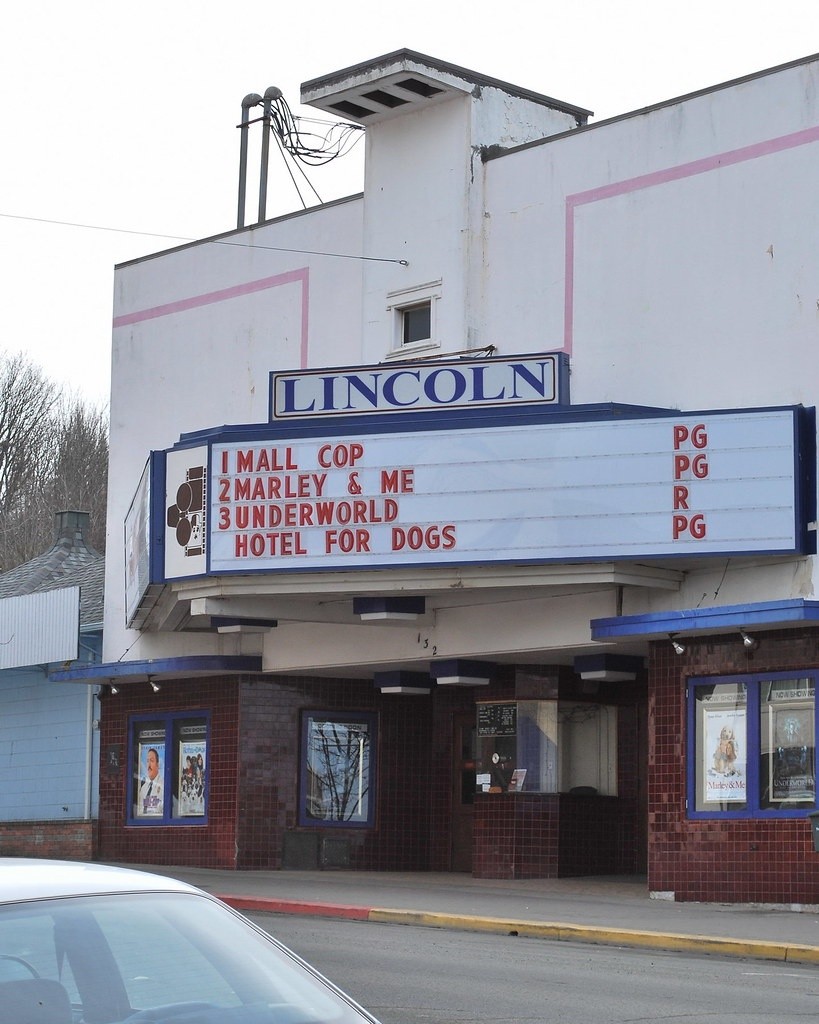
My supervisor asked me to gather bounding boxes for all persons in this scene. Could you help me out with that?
[777,722,806,774]
[724,742,743,777]
[140,748,164,814]
[181,754,205,803]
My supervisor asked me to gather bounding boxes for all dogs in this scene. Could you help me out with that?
[713,726,736,774]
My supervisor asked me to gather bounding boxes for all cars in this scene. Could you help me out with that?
[1,856,383,1024]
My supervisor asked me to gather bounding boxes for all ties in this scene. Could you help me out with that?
[143,782,153,814]
